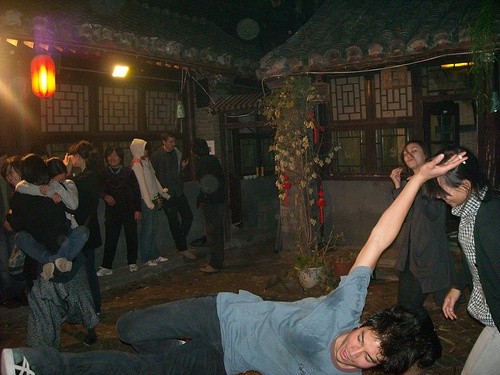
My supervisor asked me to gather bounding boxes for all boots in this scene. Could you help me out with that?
[417,312,442,370]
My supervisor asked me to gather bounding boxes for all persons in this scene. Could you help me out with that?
[150,130,197,259]
[426,151,500,375]
[3,150,470,375]
[0,140,102,352]
[97,146,142,275]
[191,138,224,274]
[390,139,464,369]
[130,139,170,266]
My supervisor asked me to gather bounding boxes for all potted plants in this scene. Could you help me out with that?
[291,252,331,292]
[325,248,357,275]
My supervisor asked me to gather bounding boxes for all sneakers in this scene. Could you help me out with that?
[129,263,138,272]
[96,265,113,276]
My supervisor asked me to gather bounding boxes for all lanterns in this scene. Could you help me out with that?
[31,54,56,101]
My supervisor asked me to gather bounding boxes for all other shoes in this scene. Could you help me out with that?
[82,333,99,347]
[5,289,29,309]
[55,257,73,272]
[40,263,55,282]
[191,235,217,248]
[0,348,36,375]
[177,249,196,259]
[142,260,157,266]
[199,263,220,273]
[154,256,169,262]
